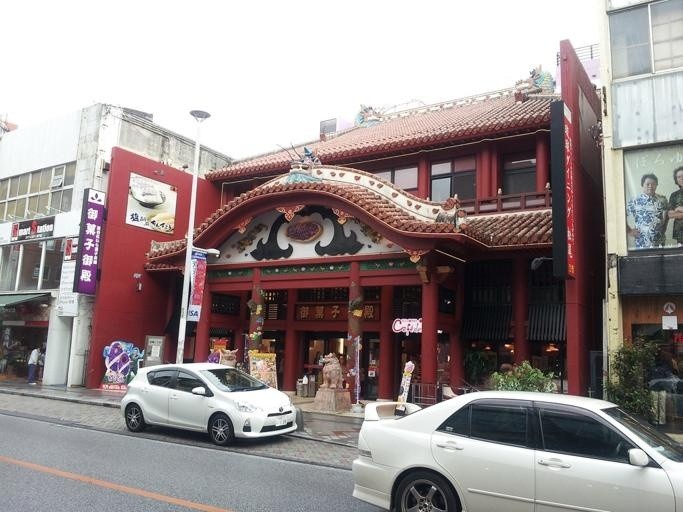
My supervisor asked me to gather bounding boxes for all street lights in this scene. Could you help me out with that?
[175,110,221,365]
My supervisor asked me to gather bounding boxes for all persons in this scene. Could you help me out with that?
[27,345,40,385]
[38,349,46,381]
[667,167,683,245]
[626,174,669,248]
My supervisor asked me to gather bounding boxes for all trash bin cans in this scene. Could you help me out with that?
[648,388,666,425]
[297,379,308,397]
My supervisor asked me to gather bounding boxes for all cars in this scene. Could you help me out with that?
[351,390,683,512]
[643,377,683,419]
[120,362,297,447]
